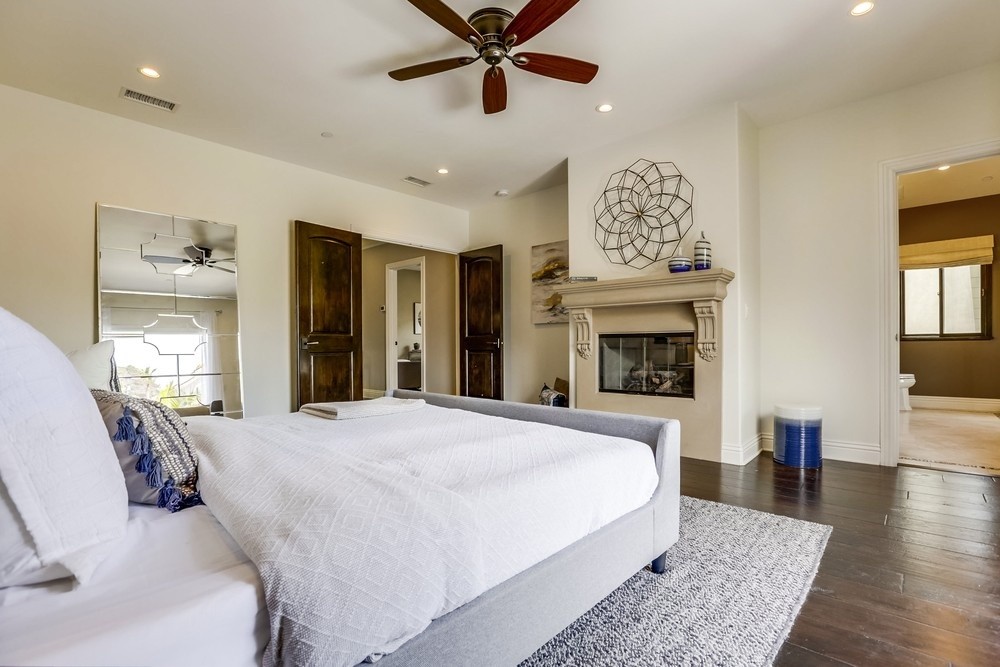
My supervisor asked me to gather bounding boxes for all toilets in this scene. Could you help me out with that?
[899,373,916,411]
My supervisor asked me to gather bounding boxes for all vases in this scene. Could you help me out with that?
[694,231,711,270]
[668,247,693,273]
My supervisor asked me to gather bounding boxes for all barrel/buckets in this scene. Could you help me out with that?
[774,404,823,468]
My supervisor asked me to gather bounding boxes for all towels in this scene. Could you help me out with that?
[298,396,427,419]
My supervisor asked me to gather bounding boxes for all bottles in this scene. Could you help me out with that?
[668,247,693,273]
[694,231,712,271]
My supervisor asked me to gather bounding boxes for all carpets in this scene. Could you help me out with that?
[516,496,832,667]
[898,407,1000,478]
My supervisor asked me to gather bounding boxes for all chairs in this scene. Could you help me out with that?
[554,377,569,397]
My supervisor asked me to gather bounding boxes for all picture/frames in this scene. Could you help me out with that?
[414,302,421,335]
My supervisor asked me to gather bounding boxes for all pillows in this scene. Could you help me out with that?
[0,304,127,588]
[65,340,121,393]
[540,382,566,407]
[89,389,199,512]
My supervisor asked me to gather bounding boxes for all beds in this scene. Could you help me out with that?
[0,304,681,667]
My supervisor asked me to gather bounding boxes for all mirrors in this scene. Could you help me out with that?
[96,202,244,419]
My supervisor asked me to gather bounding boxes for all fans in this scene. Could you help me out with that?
[142,246,236,275]
[388,0,599,114]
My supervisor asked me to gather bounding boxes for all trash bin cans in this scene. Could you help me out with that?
[773,401,823,468]
